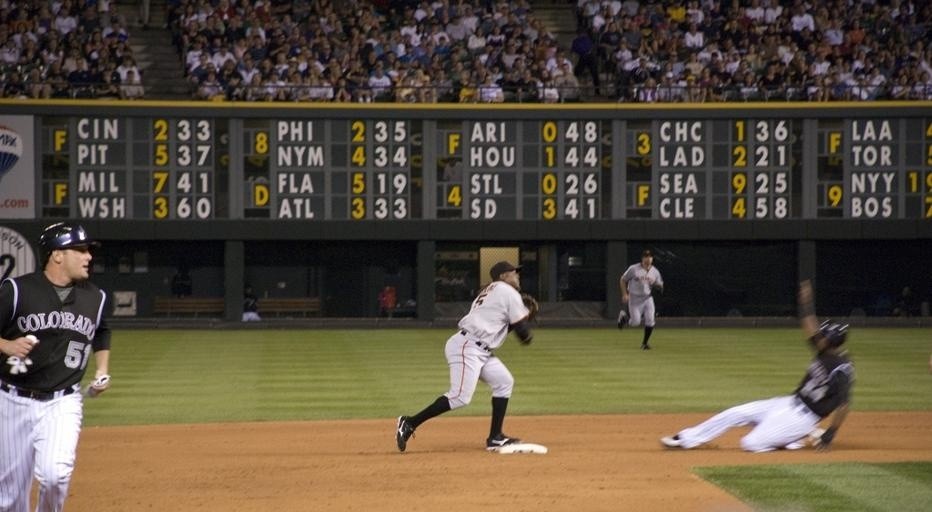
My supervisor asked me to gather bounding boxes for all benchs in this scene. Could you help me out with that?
[152,296,320,318]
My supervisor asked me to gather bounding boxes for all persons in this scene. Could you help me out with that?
[660,279,857,454]
[163,2,600,103]
[377,284,397,318]
[571,1,931,102]
[241,285,261,322]
[172,272,193,299]
[617,249,664,350]
[394,261,541,454]
[1,220,113,512]
[887,286,921,317]
[1,225,36,291]
[0,1,150,100]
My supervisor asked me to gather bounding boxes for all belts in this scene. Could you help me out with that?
[0,380,79,402]
[461,330,491,352]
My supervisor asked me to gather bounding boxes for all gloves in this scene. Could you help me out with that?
[8,334,40,375]
[84,376,110,397]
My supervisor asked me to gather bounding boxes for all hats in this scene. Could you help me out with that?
[490,261,524,280]
[642,249,653,257]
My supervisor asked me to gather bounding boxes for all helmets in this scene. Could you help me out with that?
[819,319,850,348]
[40,222,100,252]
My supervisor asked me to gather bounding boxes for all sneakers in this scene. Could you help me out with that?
[485,432,519,451]
[617,310,626,329]
[397,414,414,451]
[661,435,682,447]
